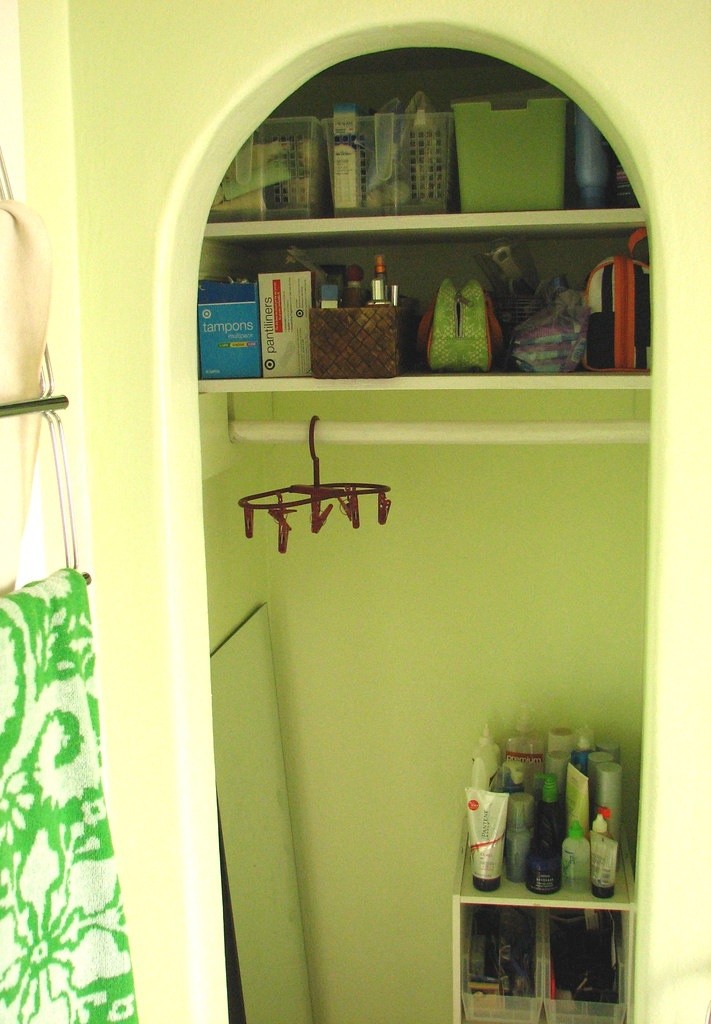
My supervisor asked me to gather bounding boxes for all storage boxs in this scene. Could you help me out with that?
[205,115,321,220]
[452,98,574,214]
[259,270,317,379]
[308,299,421,378]
[461,905,627,1023]
[318,110,456,220]
[196,280,264,380]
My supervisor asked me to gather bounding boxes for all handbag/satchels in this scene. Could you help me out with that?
[586,228,650,371]
[417,278,502,373]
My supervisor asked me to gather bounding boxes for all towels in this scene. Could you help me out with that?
[3,569,137,1024]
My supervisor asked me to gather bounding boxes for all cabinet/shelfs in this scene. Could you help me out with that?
[451,813,635,1024]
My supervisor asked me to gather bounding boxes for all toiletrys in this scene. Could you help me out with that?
[320,256,398,308]
[574,102,612,207]
[464,699,622,898]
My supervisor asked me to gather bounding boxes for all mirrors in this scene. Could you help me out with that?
[145,20,680,1024]
